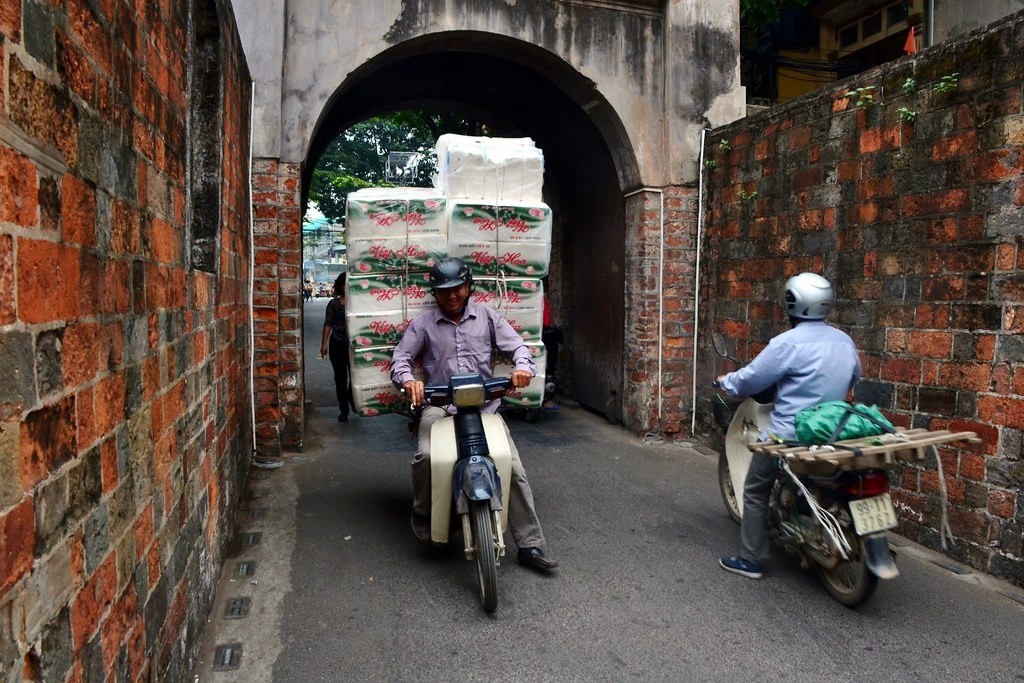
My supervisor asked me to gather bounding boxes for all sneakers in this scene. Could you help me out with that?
[718,556,763,579]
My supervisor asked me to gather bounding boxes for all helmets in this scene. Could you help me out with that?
[783,272,834,319]
[430,257,471,291]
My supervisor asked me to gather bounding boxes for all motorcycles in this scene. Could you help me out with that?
[391,369,537,612]
[702,328,900,610]
[526,325,565,424]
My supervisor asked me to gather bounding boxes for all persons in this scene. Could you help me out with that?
[389,257,560,570]
[541,281,560,410]
[320,271,358,424]
[715,270,862,577]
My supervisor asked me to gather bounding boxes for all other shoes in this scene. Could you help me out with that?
[338,411,349,422]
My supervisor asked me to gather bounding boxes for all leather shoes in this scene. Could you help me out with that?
[517,547,558,569]
[411,498,431,539]
